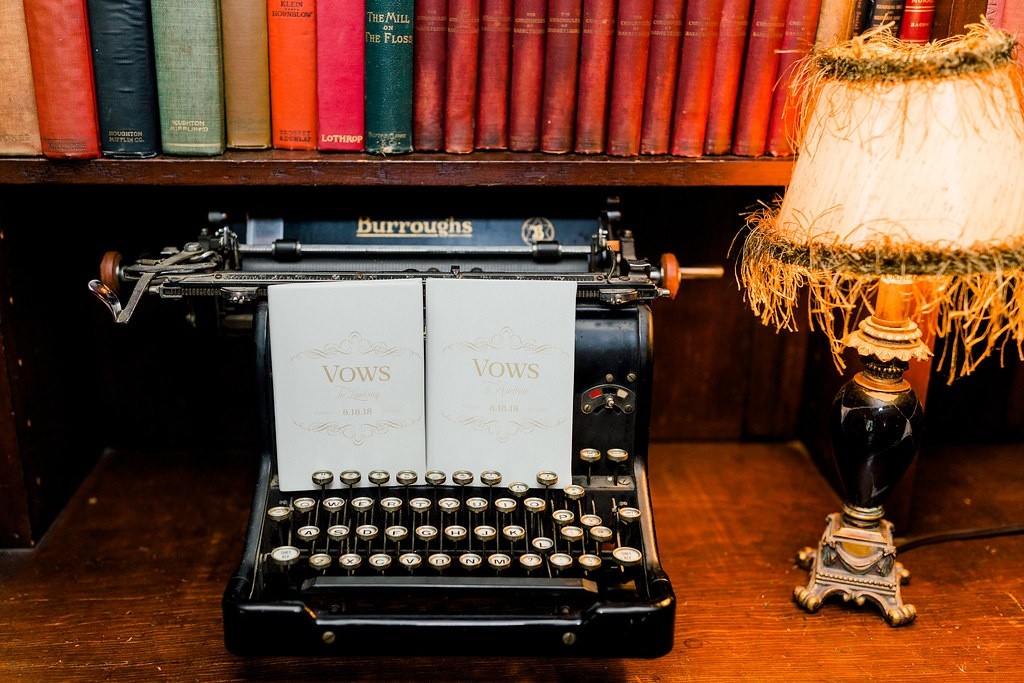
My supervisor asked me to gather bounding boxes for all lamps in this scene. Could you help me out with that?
[727,13,1024,628]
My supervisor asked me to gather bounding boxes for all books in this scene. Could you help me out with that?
[0,2,1024,160]
